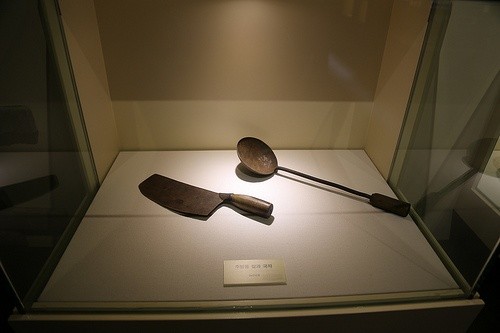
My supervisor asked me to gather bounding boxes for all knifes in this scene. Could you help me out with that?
[138,174,273,219]
